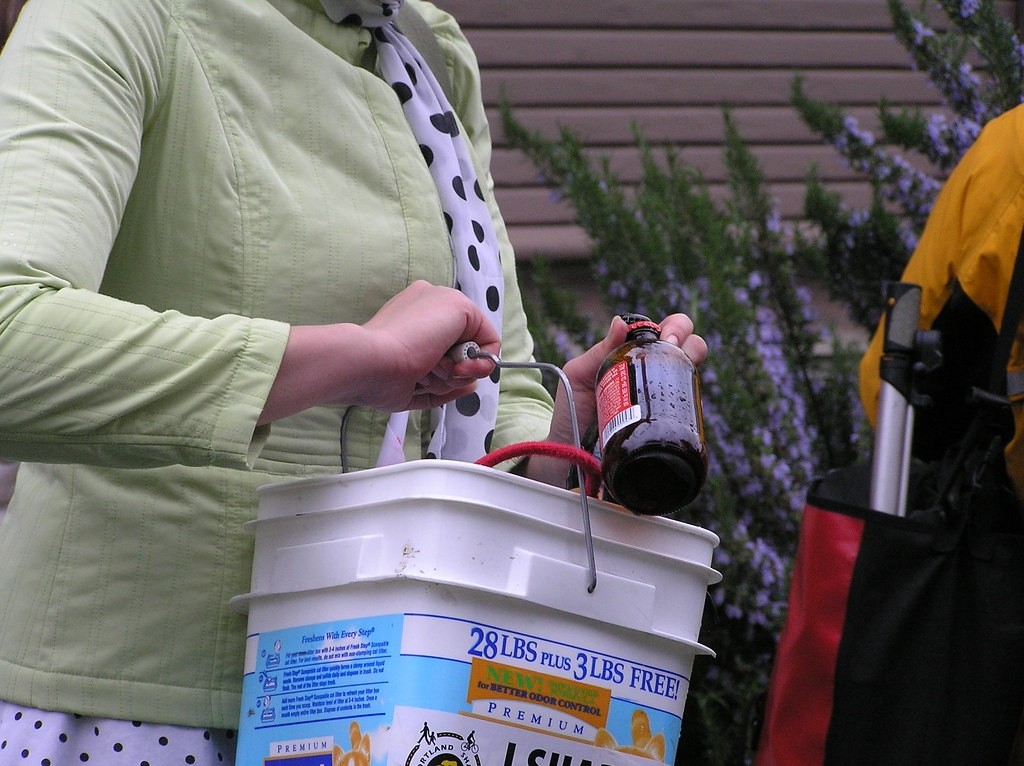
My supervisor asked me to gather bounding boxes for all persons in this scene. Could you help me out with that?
[0,0,708,766]
[857,105,1024,501]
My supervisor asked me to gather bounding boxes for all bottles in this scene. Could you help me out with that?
[594,311,708,517]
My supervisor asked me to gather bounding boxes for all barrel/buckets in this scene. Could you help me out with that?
[227,341,723,766]
